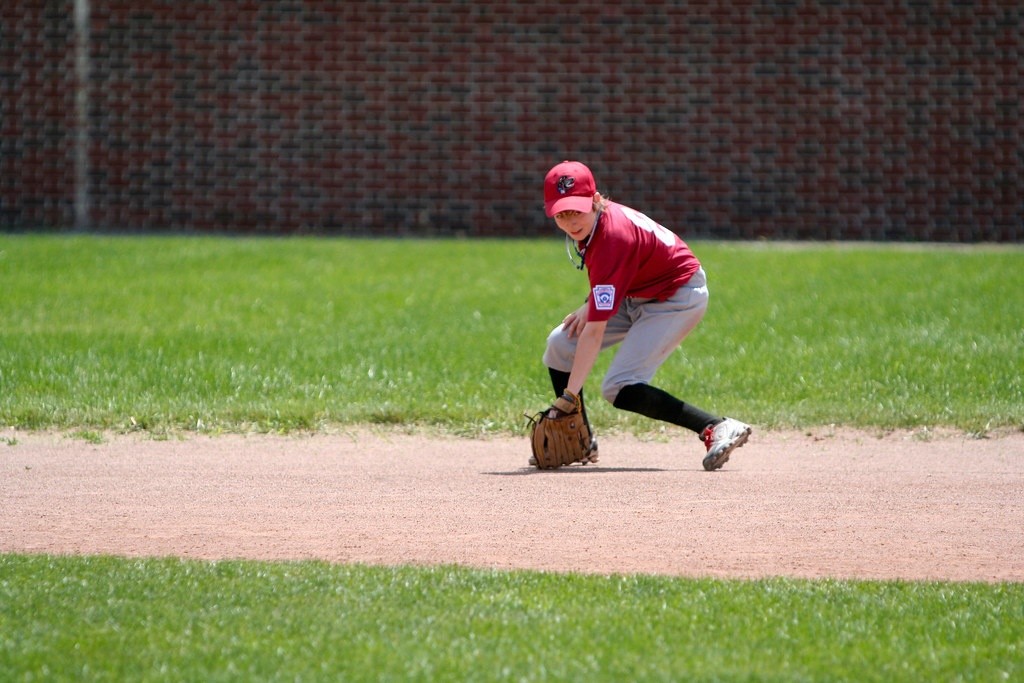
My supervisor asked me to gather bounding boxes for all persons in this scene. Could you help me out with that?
[529,160,752,471]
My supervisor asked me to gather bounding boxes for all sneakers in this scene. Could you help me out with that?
[699,416,752,470]
[528,433,599,466]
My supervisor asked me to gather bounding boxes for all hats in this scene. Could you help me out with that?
[545,161,596,217]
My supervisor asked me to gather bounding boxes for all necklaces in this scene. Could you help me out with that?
[565,199,600,270]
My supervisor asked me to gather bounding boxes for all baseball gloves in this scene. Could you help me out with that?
[529,387,600,471]
